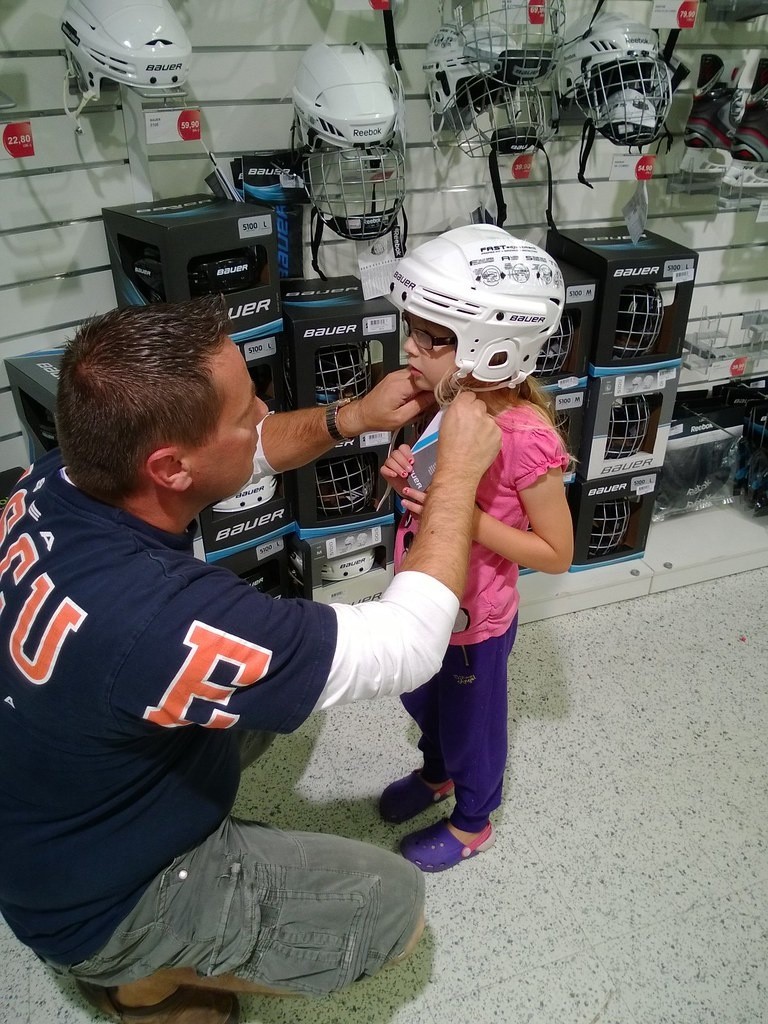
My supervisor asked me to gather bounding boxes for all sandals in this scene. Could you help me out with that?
[379,768,456,824]
[400,814,494,874]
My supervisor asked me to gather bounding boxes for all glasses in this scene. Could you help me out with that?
[402,313,457,350]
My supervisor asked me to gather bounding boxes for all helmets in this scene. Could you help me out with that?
[550,10,658,98]
[212,472,277,513]
[287,547,374,581]
[59,0,192,100]
[421,21,521,114]
[390,224,566,383]
[595,89,657,138]
[292,40,400,152]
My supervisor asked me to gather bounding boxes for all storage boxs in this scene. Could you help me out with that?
[545,225,700,377]
[100,193,282,342]
[575,370,681,481]
[281,276,400,408]
[282,521,398,605]
[201,470,296,565]
[3,341,71,467]
[232,325,281,410]
[214,534,290,599]
[289,424,395,541]
[534,375,586,472]
[566,468,662,574]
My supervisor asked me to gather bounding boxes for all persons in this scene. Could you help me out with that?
[378,223,574,872]
[1,292,501,1024]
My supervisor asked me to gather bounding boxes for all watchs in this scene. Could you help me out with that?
[323,397,358,443]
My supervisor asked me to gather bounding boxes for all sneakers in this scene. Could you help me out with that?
[678,53,746,172]
[720,58,768,187]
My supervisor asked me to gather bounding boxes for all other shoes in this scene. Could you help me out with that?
[74,975,239,1024]
